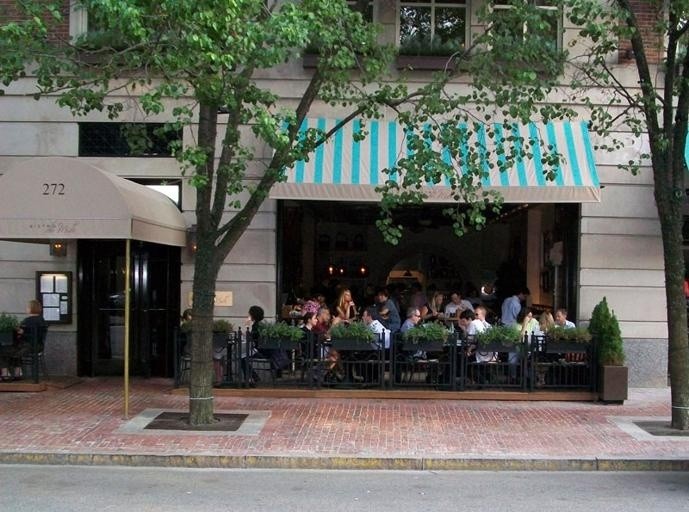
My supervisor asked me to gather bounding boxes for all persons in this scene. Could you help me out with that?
[249,287,576,389]
[180,309,193,322]
[0,301,47,383]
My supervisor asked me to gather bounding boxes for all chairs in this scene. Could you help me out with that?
[171,324,591,391]
[6,321,48,380]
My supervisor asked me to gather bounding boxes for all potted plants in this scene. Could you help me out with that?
[587,296,627,403]
[0,313,20,346]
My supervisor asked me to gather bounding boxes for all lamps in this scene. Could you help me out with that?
[49,238,67,256]
[186,223,199,255]
